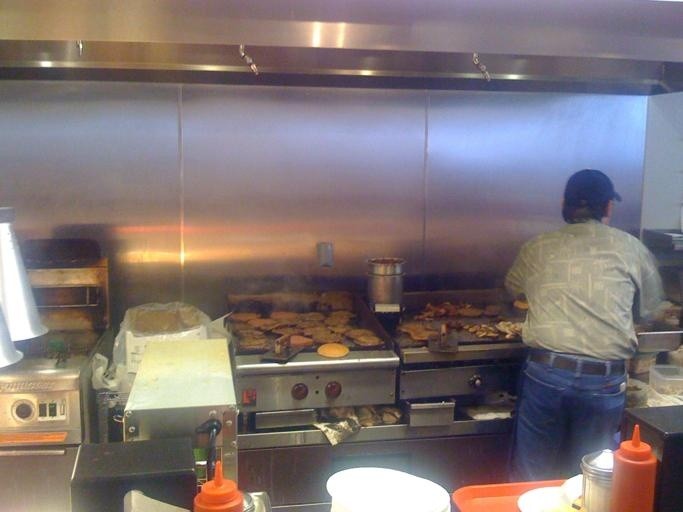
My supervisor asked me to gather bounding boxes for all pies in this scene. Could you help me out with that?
[230,306,383,350]
[397,302,488,346]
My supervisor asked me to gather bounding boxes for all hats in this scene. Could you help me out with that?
[565,170,621,203]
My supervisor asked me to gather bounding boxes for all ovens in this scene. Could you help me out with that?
[15,238,109,356]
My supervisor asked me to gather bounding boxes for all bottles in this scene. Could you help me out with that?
[193,461,243,512]
[611,425,658,512]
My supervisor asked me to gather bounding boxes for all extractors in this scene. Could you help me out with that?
[2,39,681,95]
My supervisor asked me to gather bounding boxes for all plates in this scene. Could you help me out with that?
[517,486,566,512]
[326,468,452,511]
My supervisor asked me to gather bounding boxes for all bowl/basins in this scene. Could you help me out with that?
[581,450,613,511]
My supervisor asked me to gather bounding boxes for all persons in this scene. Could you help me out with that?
[500,169,665,483]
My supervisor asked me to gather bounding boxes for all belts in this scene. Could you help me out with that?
[532,352,625,376]
